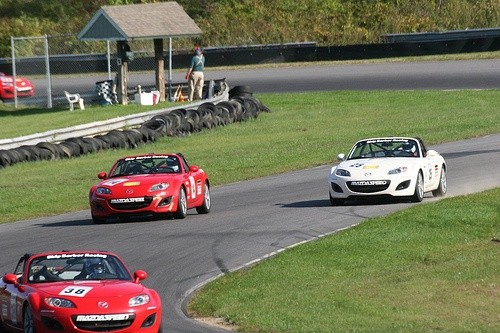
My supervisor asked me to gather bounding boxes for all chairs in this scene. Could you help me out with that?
[64,90,85,111]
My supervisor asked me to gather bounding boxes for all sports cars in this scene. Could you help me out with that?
[89,152,212,224]
[0,247,162,333]
[0,71,34,103]
[328,135,447,206]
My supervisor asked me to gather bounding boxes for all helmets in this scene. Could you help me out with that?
[85,257,104,274]
[166,156,179,172]
[401,142,416,153]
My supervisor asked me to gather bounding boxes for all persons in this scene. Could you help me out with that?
[86,259,105,280]
[160,157,180,173]
[402,142,417,156]
[185,50,205,102]
